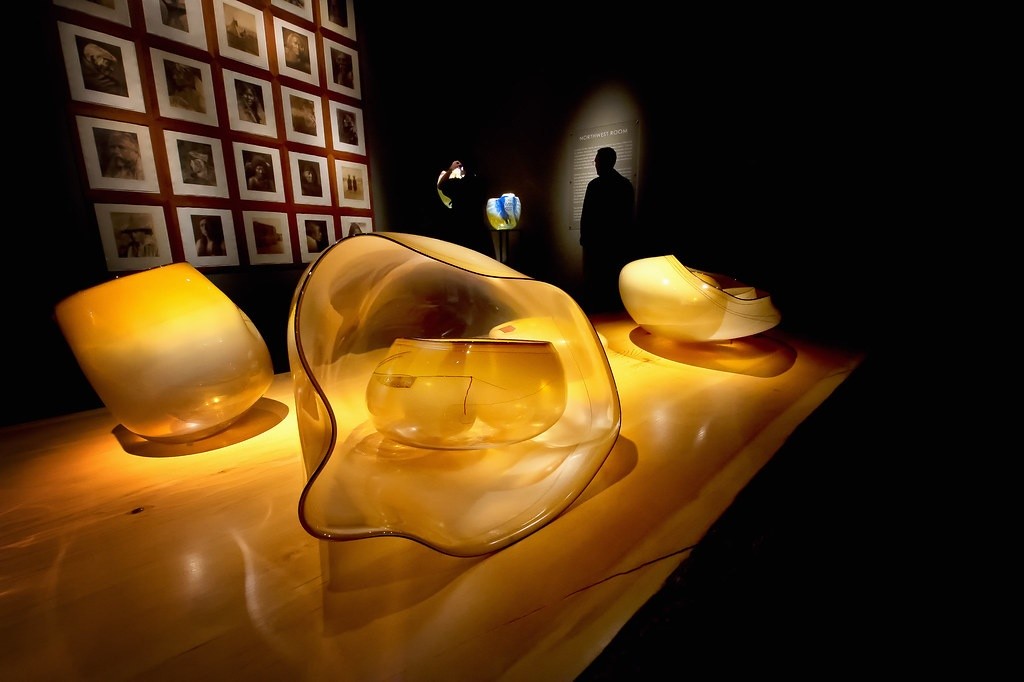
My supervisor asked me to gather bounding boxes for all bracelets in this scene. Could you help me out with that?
[448,169,452,173]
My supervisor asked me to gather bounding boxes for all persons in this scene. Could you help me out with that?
[436,159,493,260]
[83,32,363,261]
[579,146,636,313]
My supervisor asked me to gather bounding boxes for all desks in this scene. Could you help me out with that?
[0,314,867,682]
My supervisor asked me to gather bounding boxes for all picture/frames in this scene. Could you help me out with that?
[47,0,378,276]
